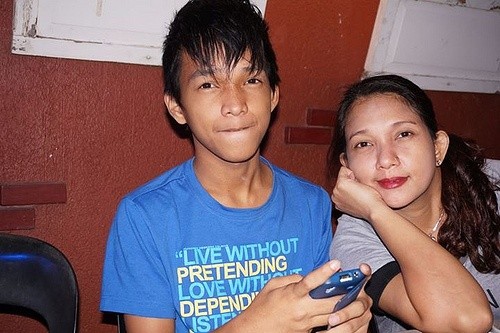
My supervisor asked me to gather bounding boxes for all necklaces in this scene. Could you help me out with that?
[427,207,444,241]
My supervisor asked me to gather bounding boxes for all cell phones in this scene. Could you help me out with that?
[309,268,365,300]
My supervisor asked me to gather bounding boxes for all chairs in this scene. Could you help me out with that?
[0,233,80,333]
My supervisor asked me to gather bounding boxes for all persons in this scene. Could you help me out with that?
[98,0,373,333]
[328,74,500,333]
